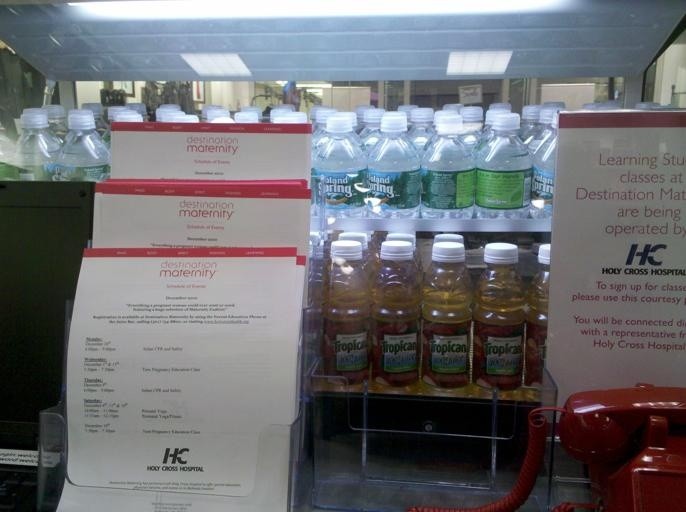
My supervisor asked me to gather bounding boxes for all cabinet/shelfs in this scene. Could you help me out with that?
[314,206,551,407]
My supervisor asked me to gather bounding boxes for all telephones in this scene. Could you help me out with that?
[559,383,686,512]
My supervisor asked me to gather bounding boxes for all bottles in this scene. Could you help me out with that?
[10,100,681,221]
[324,230,551,403]
